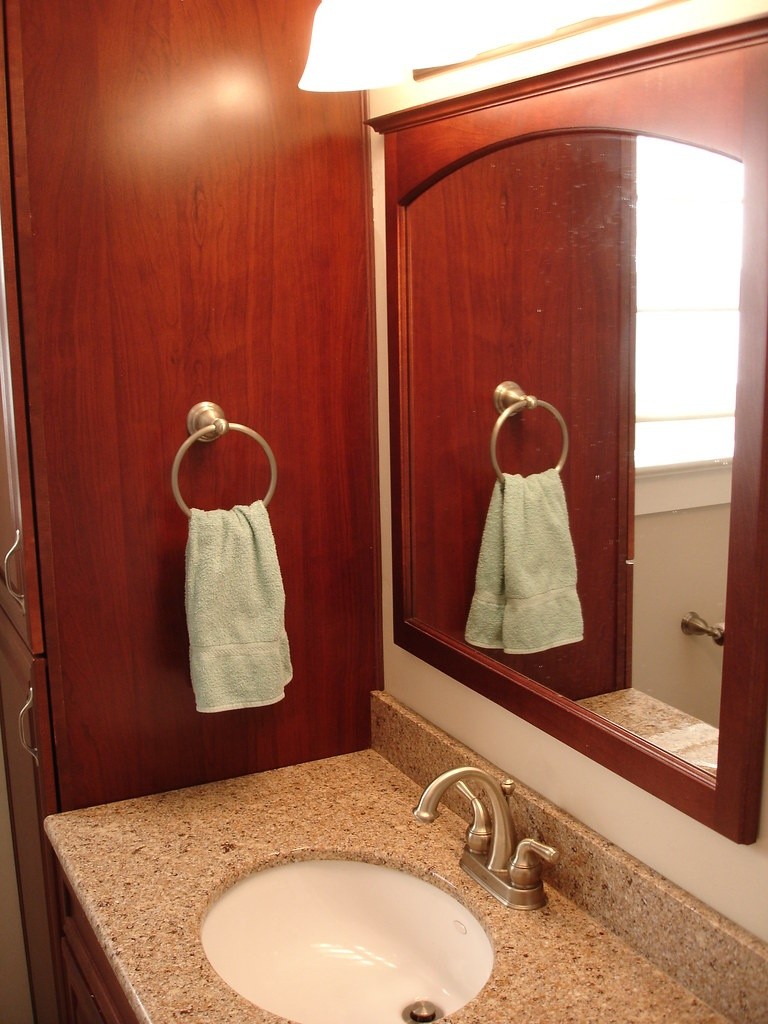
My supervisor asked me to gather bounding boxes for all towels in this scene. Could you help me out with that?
[463,467,586,655]
[183,497,295,716]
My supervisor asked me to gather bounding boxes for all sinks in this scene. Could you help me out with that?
[199,847,497,1024]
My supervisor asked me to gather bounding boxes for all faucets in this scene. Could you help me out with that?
[411,766,517,873]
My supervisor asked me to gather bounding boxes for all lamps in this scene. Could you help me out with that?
[298,0,415,94]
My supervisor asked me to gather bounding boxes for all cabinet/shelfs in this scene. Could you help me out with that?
[0,0,383,1024]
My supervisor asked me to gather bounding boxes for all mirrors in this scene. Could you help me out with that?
[361,16,767,845]
